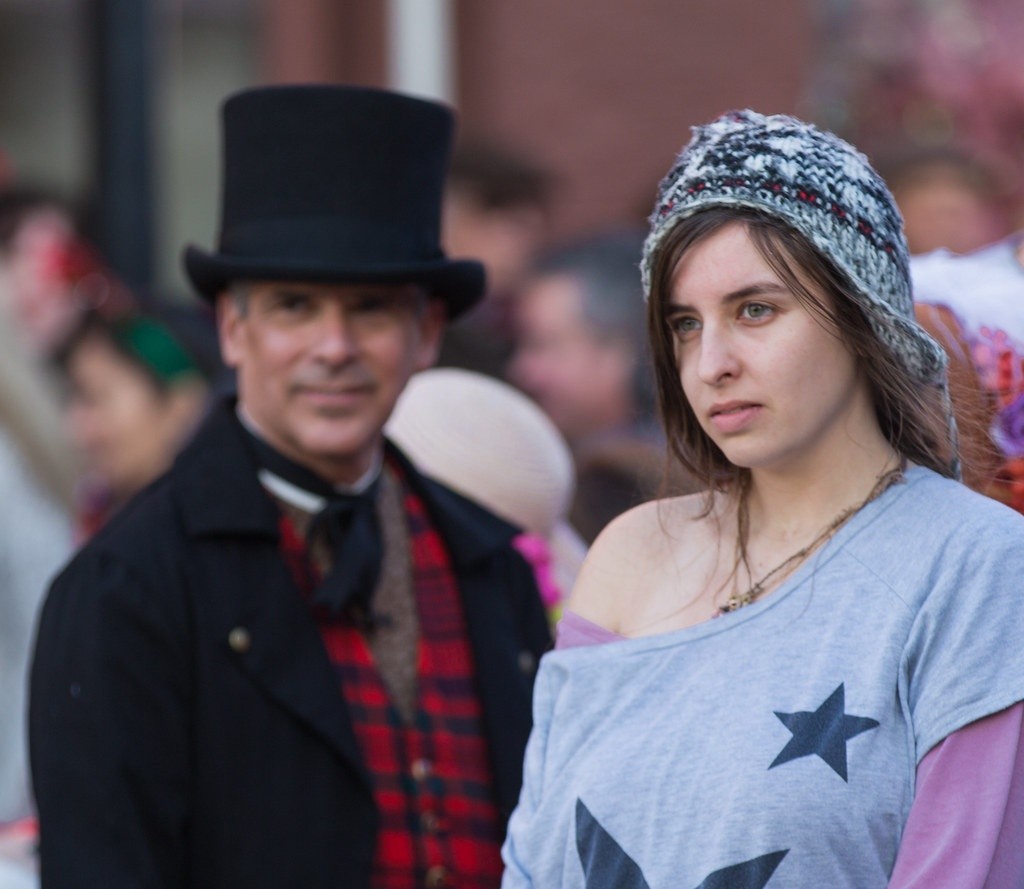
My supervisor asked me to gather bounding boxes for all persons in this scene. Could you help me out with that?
[497,105,1023,889]
[16,85,555,889]
[878,146,1022,520]
[0,183,238,889]
[373,142,708,633]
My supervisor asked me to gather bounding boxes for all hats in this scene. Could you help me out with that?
[185,86,487,323]
[382,366,589,614]
[641,110,964,485]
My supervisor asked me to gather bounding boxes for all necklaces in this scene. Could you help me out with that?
[709,442,909,621]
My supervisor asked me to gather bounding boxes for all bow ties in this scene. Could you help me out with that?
[239,422,385,628]
